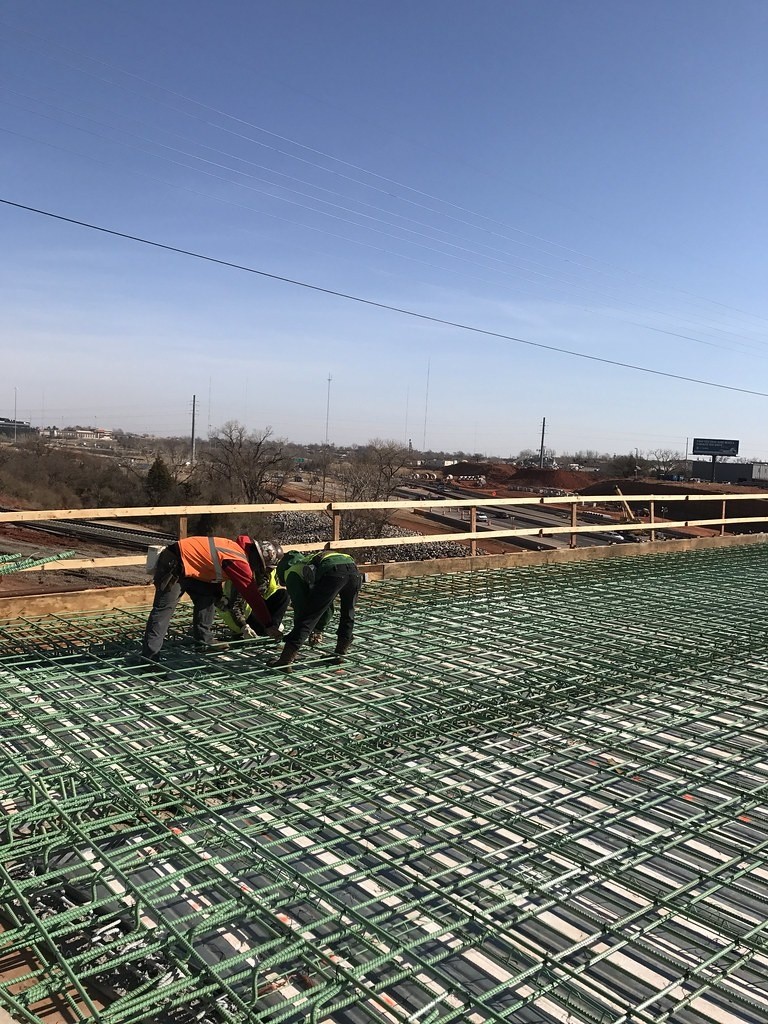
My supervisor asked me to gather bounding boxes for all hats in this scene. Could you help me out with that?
[276,549,306,587]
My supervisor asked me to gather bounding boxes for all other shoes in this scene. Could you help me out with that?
[139,652,168,664]
[195,638,230,653]
[231,635,245,649]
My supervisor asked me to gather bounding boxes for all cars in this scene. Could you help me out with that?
[599,531,640,543]
[476,511,487,522]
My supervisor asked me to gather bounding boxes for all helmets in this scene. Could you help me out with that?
[253,539,277,570]
[269,541,284,568]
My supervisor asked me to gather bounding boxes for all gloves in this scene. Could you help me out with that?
[213,594,230,611]
[230,598,252,627]
[265,627,284,643]
[241,624,258,639]
[308,632,323,645]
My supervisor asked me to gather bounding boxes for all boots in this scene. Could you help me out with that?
[266,647,298,673]
[320,643,350,665]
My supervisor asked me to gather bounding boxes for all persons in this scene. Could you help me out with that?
[139,535,291,664]
[266,549,362,675]
